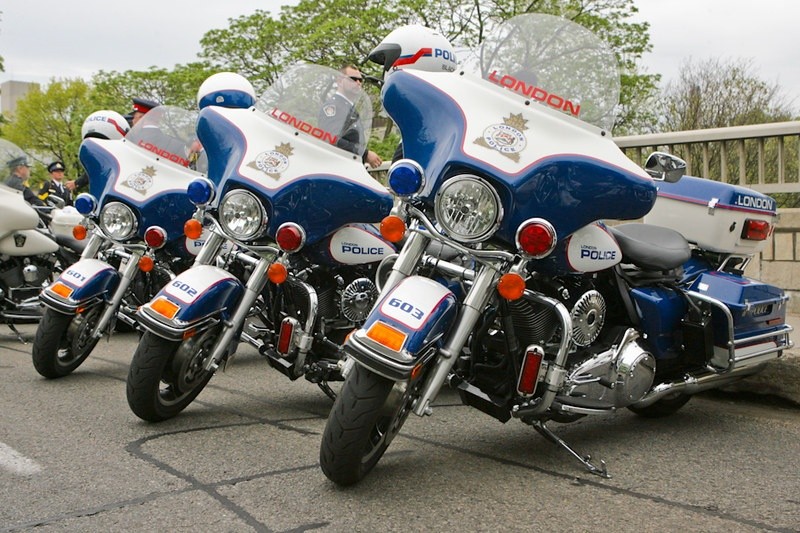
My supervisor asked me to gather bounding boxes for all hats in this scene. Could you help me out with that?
[48,161,65,172]
[127,96,159,115]
[6,155,33,169]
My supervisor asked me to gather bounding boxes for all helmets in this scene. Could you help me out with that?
[368,26,457,87]
[80,110,130,139]
[196,70,256,111]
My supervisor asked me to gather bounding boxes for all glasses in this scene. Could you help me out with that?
[349,76,364,84]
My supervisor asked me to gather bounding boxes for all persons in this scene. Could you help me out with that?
[318,64,382,169]
[391,139,411,228]
[3,155,89,229]
[123,99,209,173]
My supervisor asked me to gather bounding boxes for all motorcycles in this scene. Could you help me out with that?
[315,13,797,489]
[0,60,419,425]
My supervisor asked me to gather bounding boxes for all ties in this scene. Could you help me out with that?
[59,184,61,192]
